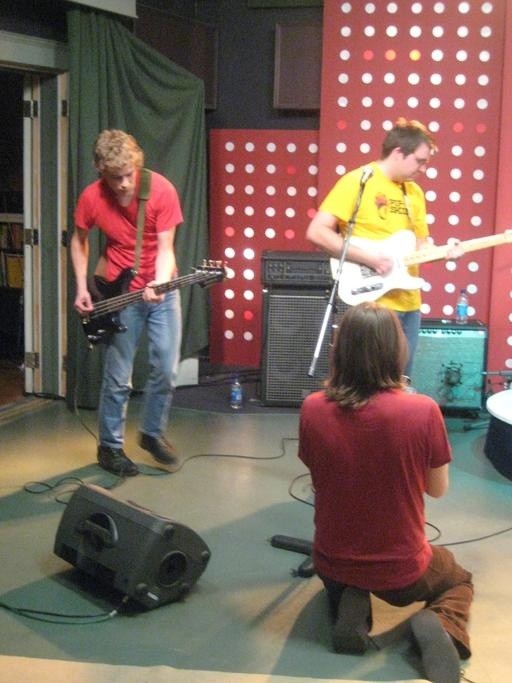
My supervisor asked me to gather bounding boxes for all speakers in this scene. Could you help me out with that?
[53,480,213,609]
[259,287,351,409]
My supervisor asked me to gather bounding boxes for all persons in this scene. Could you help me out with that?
[69,127,188,478]
[305,116,467,379]
[295,300,475,683]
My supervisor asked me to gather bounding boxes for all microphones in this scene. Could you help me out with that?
[357,163,376,189]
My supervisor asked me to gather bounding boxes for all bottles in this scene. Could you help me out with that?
[454,288,470,325]
[229,378,244,410]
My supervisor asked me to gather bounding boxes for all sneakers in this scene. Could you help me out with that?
[140,432,179,464]
[332,585,368,655]
[96,445,138,477]
[409,608,462,683]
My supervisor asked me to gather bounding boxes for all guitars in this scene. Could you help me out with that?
[330,229,512,305]
[82,258,228,345]
